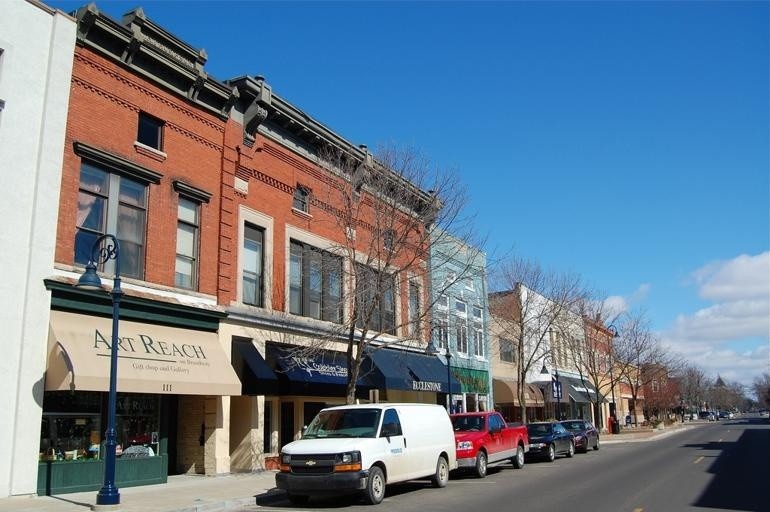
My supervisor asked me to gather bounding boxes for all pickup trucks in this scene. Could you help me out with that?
[446,409,532,480]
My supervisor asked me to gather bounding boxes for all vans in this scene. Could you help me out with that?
[41,410,127,455]
[272,402,456,510]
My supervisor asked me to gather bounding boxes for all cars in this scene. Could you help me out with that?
[523,421,575,465]
[682,409,735,422]
[562,420,601,453]
[759,409,770,416]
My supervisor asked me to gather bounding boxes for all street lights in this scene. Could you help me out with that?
[608,323,622,431]
[71,232,122,506]
[428,325,454,412]
[538,356,561,421]
